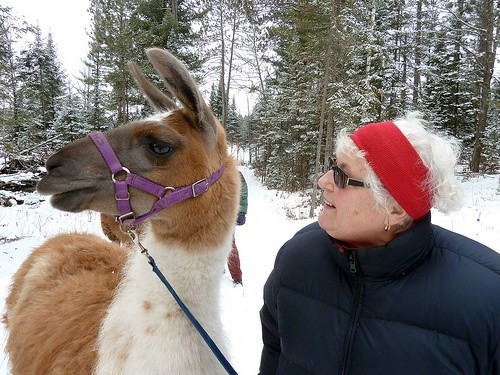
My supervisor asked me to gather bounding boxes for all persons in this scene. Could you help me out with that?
[257,110,500,375]
[226,171,248,298]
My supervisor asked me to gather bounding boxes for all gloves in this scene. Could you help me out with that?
[237,212,245,225]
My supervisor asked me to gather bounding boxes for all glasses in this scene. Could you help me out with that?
[326,157,387,192]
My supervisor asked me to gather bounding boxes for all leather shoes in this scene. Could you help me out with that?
[234,277,243,285]
